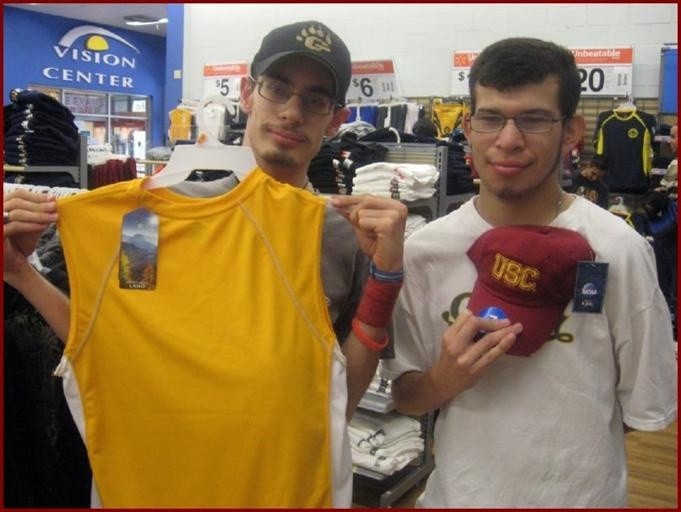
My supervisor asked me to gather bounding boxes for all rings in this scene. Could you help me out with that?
[2,207,10,223]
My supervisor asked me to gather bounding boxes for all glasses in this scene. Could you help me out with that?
[249,72,345,116]
[469,108,573,135]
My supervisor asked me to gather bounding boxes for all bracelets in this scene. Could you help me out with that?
[352,267,404,354]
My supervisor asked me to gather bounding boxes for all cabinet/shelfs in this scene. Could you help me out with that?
[2,130,86,188]
[309,140,446,508]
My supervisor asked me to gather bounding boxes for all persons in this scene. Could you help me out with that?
[389,38,679,509]
[2,18,408,509]
[568,153,611,208]
[661,123,680,202]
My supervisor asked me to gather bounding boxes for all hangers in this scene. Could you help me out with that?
[618,93,637,113]
[143,95,354,213]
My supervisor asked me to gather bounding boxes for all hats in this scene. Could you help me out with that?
[464,225,597,358]
[250,19,354,114]
